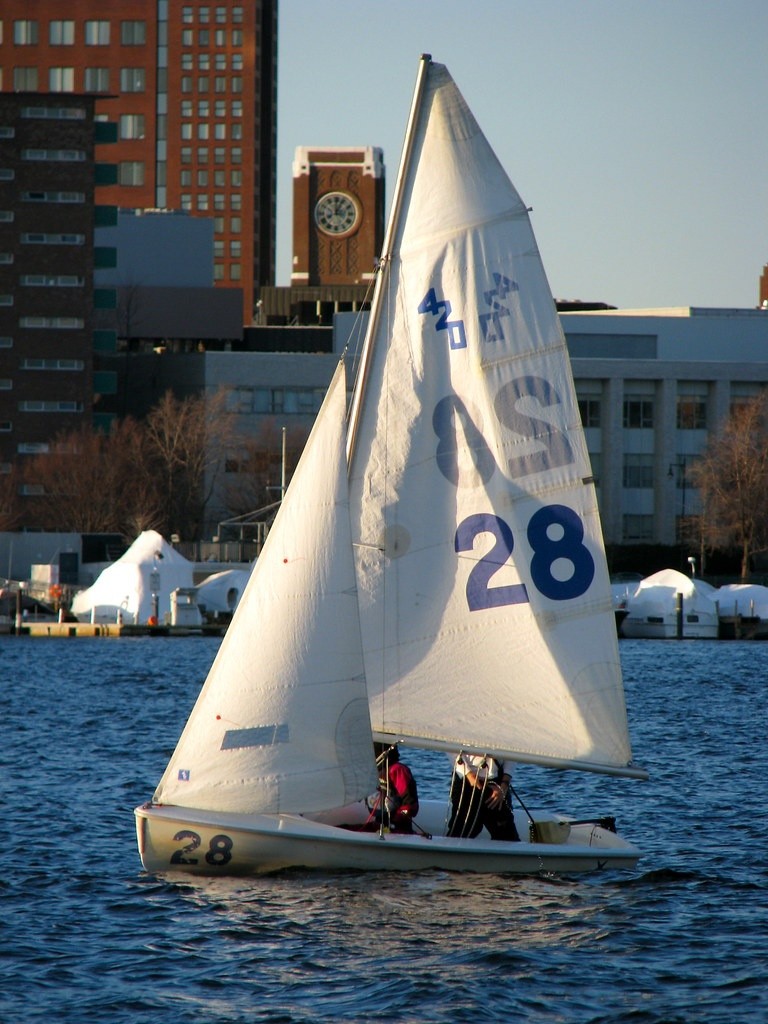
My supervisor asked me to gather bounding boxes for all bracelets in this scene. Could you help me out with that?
[502,779,510,788]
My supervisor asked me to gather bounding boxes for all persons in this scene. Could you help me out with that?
[446,751,521,842]
[366,741,419,834]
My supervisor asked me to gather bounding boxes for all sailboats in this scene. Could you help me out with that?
[129,50,652,881]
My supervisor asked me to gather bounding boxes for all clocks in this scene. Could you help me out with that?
[313,191,358,237]
